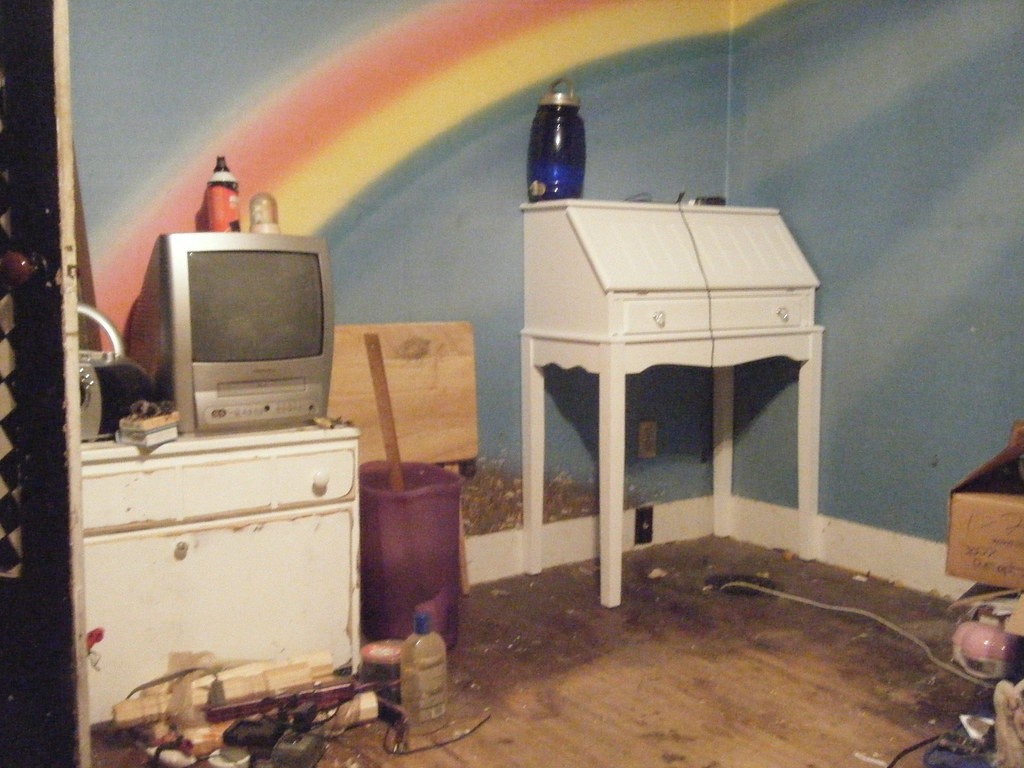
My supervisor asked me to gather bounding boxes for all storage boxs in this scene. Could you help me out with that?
[944,420,1024,591]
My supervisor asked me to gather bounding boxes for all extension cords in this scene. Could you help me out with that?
[110,656,379,758]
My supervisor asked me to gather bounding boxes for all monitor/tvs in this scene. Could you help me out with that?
[123,233,335,436]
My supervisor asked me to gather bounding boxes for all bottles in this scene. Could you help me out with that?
[527,75,585,201]
[402,613,448,734]
[205,153,242,231]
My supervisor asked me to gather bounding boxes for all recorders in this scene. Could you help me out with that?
[76,302,149,446]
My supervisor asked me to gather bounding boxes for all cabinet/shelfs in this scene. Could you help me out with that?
[519,200,826,608]
[79,425,362,734]
